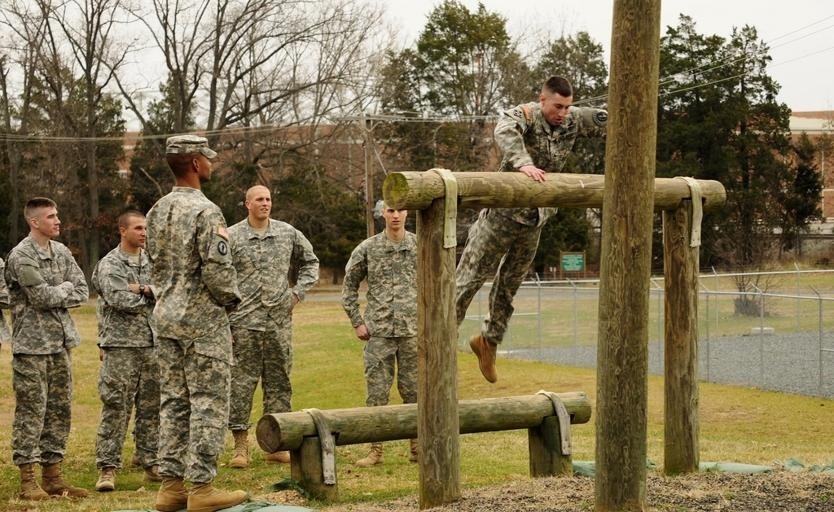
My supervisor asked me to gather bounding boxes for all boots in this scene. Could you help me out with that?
[42,462,88,499]
[230,430,251,468]
[19,463,50,501]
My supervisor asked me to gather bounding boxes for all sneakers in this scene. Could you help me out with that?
[155,477,189,512]
[187,482,248,512]
[407,439,418,463]
[146,464,165,482]
[264,450,292,463]
[355,441,386,468]
[94,466,117,491]
[468,334,499,384]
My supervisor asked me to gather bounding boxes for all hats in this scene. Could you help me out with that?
[165,134,218,159]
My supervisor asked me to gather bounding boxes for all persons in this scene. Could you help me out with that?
[131,418,142,468]
[226,185,319,469]
[3,196,88,500]
[339,202,420,467]
[91,211,165,489]
[455,78,607,382]
[145,132,249,511]
